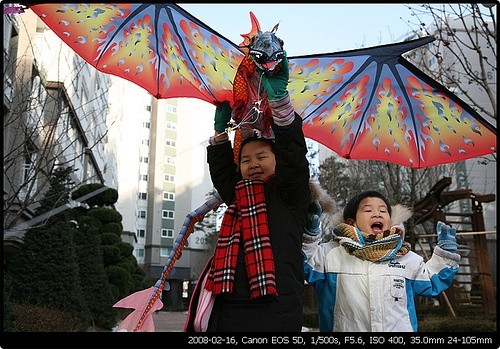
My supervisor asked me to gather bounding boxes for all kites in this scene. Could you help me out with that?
[4,1,496,334]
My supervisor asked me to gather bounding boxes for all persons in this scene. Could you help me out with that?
[302,191,461,334]
[207,52,310,334]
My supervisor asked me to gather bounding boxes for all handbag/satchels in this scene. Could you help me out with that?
[183,254,218,332]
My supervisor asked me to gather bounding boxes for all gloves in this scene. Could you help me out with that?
[305,200,323,235]
[437,221,459,252]
[256,51,290,100]
[214,100,232,132]
